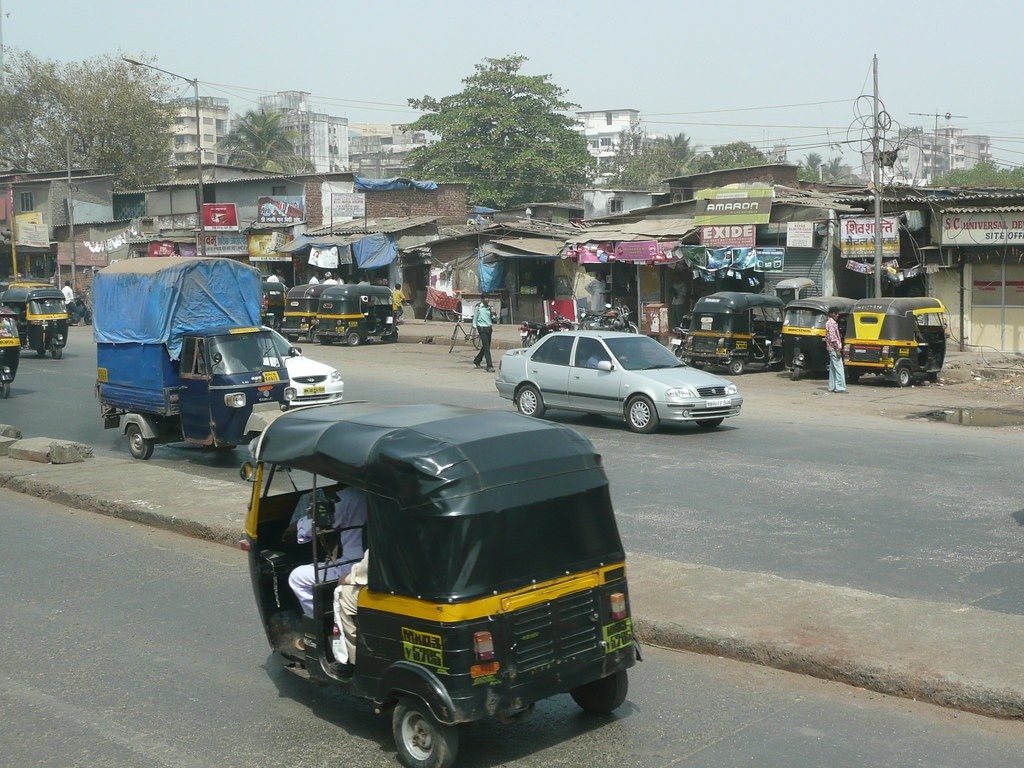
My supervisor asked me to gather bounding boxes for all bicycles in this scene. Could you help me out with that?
[423,285,460,322]
[448,309,483,353]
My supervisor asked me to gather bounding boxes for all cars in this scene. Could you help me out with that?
[495,330,744,435]
[260,325,346,412]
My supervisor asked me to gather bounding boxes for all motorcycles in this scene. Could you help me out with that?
[778,297,857,380]
[518,302,638,348]
[0,279,69,400]
[239,400,645,768]
[685,291,787,377]
[671,311,694,362]
[843,295,947,387]
[67,296,92,327]
[259,280,399,347]
[92,256,296,465]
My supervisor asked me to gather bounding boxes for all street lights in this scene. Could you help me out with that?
[120,56,205,258]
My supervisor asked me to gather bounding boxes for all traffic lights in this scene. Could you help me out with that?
[0,229,13,247]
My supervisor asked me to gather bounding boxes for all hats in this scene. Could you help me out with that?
[325,271,332,277]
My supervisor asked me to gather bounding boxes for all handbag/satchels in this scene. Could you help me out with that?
[490,307,497,324]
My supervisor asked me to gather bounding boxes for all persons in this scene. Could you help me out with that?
[61,280,77,327]
[289,479,370,627]
[584,354,602,369]
[585,273,607,313]
[672,273,688,327]
[260,269,389,318]
[473,293,497,373]
[913,323,924,355]
[316,546,369,682]
[825,307,847,393]
[392,283,406,320]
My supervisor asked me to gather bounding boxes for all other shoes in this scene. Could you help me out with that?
[474,361,480,368]
[834,389,849,394]
[322,658,355,679]
[396,318,400,323]
[295,637,306,651]
[487,366,495,372]
